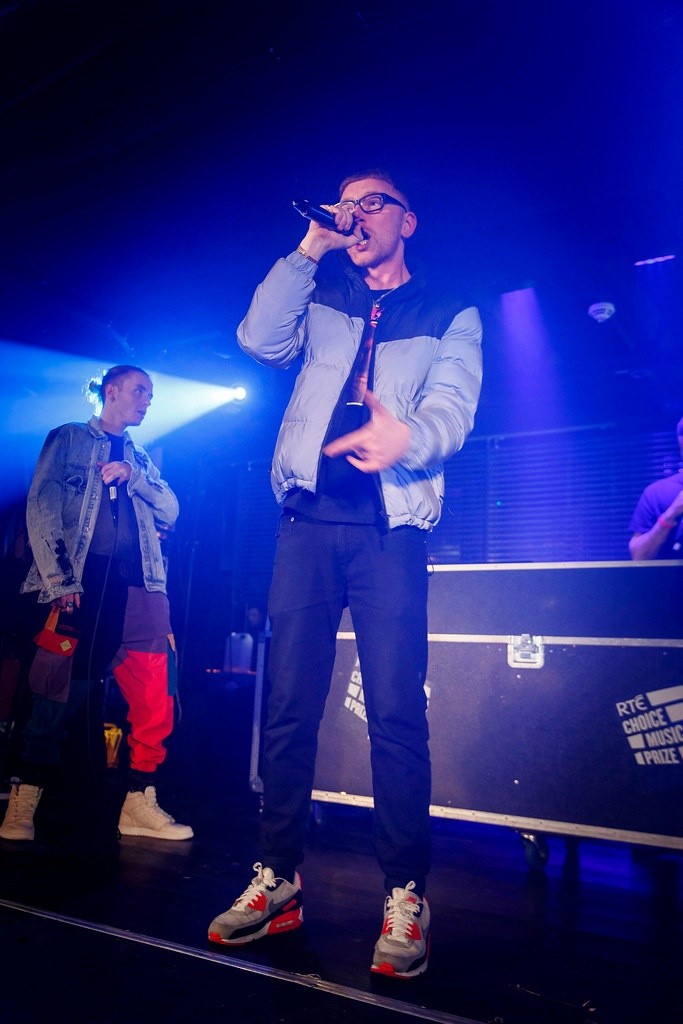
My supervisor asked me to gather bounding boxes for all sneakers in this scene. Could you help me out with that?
[370,879,434,979]
[208,863,305,945]
[0,784,43,841]
[118,785,194,841]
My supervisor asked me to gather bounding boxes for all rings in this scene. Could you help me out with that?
[67,602,74,607]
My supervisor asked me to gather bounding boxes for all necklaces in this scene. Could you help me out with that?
[371,282,405,308]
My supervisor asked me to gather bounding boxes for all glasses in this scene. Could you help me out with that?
[342,192,407,215]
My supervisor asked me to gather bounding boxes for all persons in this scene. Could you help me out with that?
[208,174,483,978]
[0,365,193,841]
[627,416,683,561]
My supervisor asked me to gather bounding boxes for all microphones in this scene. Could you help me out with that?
[107,479,119,527]
[292,199,364,238]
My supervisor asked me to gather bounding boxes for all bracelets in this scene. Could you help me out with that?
[295,246,321,266]
[658,514,675,529]
